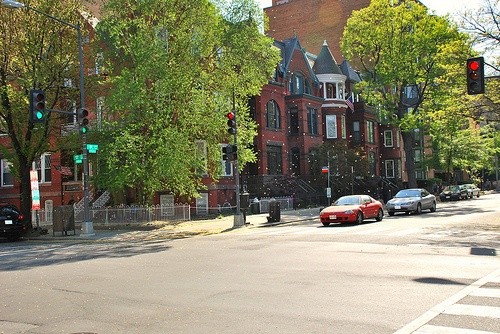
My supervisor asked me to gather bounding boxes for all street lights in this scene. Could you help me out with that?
[2,0,92,222]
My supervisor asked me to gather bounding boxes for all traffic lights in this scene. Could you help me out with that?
[466,56,485,95]
[227,111,236,135]
[223,144,236,162]
[30,89,46,123]
[78,108,89,134]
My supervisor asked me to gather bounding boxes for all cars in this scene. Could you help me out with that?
[0,202,26,241]
[439,184,480,202]
[385,189,436,216]
[320,195,384,226]
